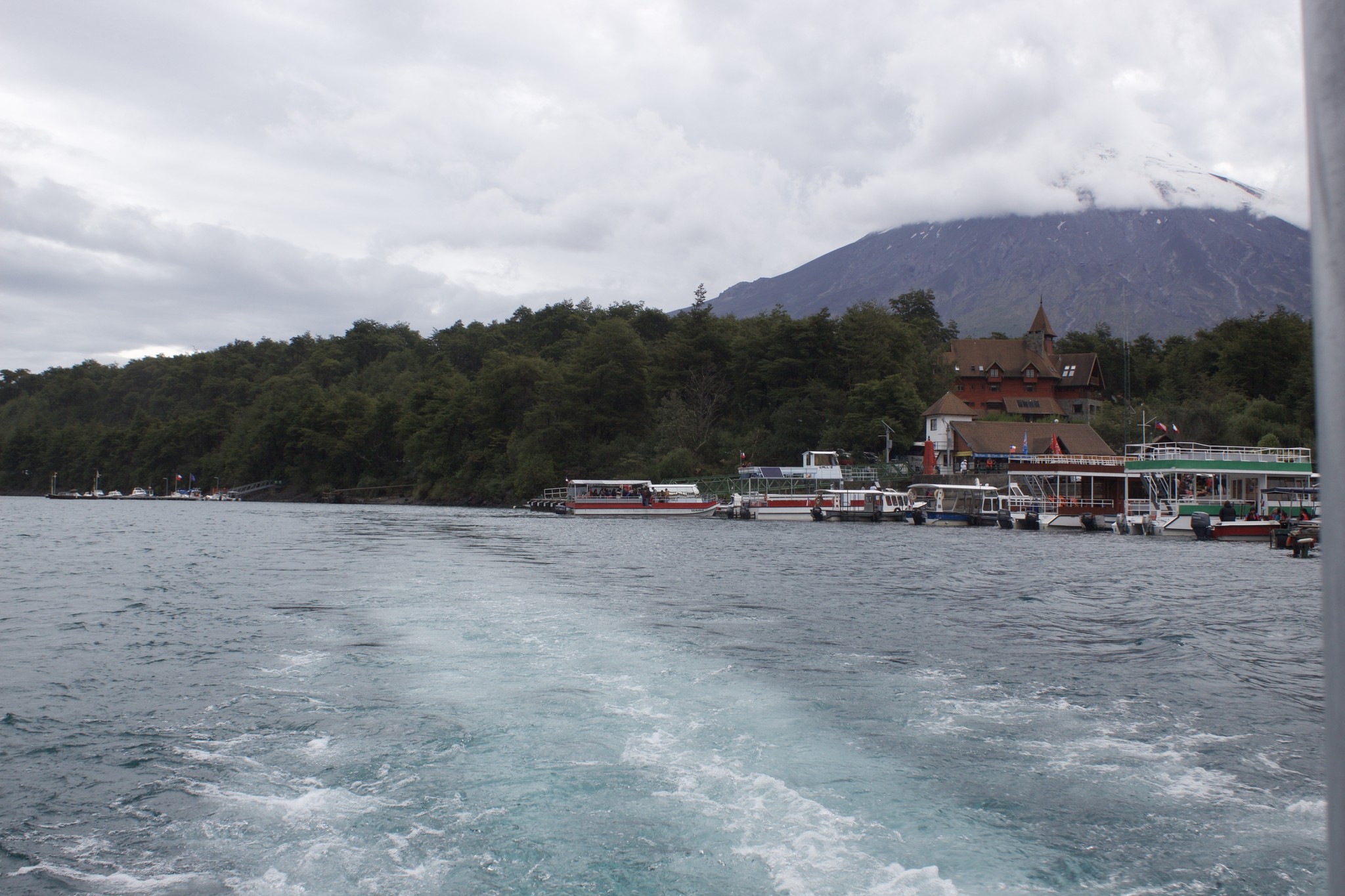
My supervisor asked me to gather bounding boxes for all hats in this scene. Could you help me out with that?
[964,460,967,461]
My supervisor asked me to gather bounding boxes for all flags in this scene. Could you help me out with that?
[566,478,569,482]
[741,452,745,457]
[1022,431,1027,454]
[1050,432,1062,454]
[1172,423,1179,433]
[1155,421,1166,432]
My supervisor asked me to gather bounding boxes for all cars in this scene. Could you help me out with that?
[837,448,882,463]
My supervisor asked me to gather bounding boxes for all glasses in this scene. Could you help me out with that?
[1250,509,1255,511]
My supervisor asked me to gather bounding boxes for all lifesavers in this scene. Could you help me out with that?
[875,510,882,519]
[1286,533,1315,549]
[583,495,592,498]
[805,473,811,478]
[907,489,917,501]
[814,496,824,507]
[831,497,840,506]
[934,489,944,501]
[894,507,901,513]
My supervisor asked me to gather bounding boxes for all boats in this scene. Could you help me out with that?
[808,489,928,522]
[1109,442,1321,543]
[1189,486,1321,549]
[724,490,904,521]
[44,487,242,501]
[997,452,1155,533]
[902,477,998,527]
[554,479,721,518]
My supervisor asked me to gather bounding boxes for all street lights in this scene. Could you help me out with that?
[214,477,219,492]
[51,476,56,494]
[163,478,168,496]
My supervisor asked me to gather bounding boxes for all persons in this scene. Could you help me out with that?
[992,459,997,473]
[742,462,754,467]
[1206,475,1227,495]
[866,466,872,476]
[960,460,967,474]
[861,483,865,490]
[926,488,931,507]
[986,458,993,474]
[870,483,878,490]
[718,498,723,505]
[592,487,669,504]
[975,460,980,474]
[886,485,890,491]
[1219,500,1311,521]
